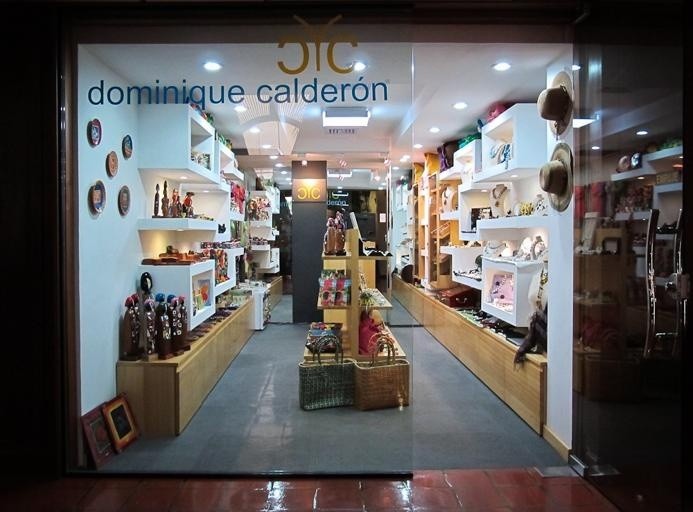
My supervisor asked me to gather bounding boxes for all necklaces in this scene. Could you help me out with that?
[444,189,455,205]
[484,242,506,254]
[491,186,507,208]
[535,268,548,312]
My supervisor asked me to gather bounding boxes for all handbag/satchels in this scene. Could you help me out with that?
[353,333,409,410]
[298,335,357,410]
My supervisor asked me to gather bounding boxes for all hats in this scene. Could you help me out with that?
[539,143,573,212]
[537,72,572,135]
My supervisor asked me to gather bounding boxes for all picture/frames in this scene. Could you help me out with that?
[99,391,140,453]
[80,403,117,467]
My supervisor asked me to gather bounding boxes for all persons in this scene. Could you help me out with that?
[488,183,510,218]
[441,185,458,212]
[527,258,548,352]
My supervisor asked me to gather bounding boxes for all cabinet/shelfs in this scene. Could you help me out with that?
[610,144,686,291]
[573,249,640,403]
[394,102,548,330]
[117,293,255,437]
[138,103,284,332]
[304,229,407,363]
